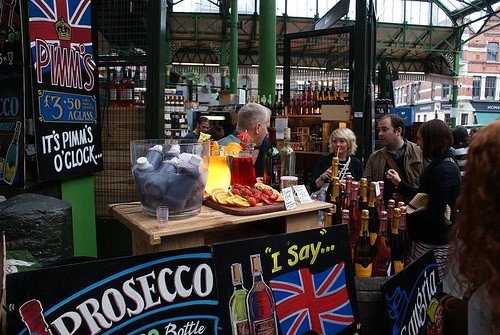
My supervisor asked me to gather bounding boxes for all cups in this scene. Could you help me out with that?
[129,138,211,219]
[281,176,298,196]
[156,205,170,227]
[227,150,257,190]
[202,156,231,193]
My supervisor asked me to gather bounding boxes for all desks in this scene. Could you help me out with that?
[108,200,333,256]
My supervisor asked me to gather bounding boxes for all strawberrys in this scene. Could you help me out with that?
[230,184,279,207]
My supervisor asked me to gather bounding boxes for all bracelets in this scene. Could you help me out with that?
[320,174,326,181]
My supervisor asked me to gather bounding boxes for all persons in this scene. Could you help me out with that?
[361,114,482,198]
[181,103,271,178]
[197,73,220,103]
[310,128,362,193]
[421,119,500,335]
[384,118,462,283]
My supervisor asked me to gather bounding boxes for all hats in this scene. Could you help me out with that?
[403,193,452,224]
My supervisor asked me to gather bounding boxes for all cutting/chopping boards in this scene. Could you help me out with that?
[203,199,286,215]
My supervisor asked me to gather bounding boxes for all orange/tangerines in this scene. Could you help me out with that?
[211,188,250,207]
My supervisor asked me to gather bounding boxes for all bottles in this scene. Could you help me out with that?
[3,121,22,185]
[280,127,296,177]
[164,95,190,139]
[132,143,203,210]
[109,67,144,107]
[263,127,282,192]
[326,156,408,277]
[291,126,323,152]
[207,103,244,112]
[246,79,351,116]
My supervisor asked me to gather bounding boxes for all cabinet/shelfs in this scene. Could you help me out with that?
[270,115,322,154]
[165,104,189,130]
